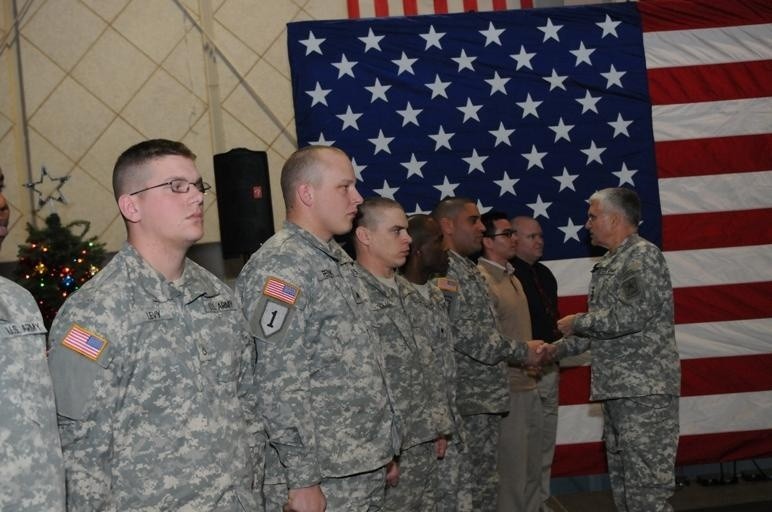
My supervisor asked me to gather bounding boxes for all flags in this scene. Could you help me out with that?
[286,0,772,480]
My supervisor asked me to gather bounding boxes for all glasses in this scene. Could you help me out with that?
[127,178,211,195]
[486,230,517,238]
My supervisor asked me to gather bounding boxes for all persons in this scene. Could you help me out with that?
[526,186,684,512]
[0,169,67,512]
[48,137,270,512]
[232,145,402,512]
[353,195,567,512]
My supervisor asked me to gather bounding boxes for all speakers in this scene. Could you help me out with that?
[213,146,275,260]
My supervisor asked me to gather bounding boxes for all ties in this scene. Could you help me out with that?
[532,266,561,342]
